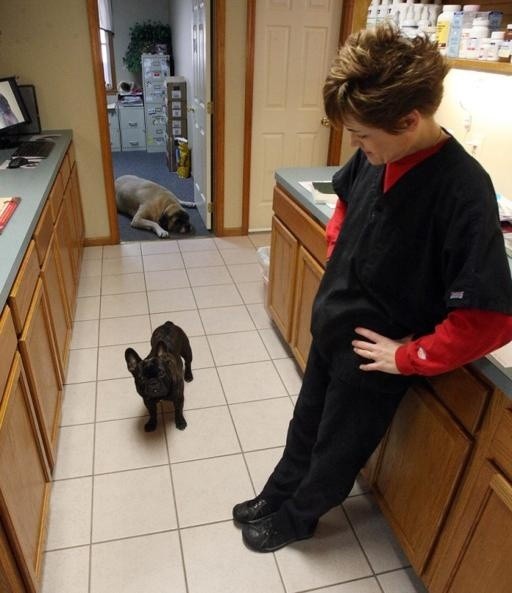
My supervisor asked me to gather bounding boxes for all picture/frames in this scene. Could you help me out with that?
[7,86,41,135]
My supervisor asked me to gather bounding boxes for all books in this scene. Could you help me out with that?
[0,196,22,234]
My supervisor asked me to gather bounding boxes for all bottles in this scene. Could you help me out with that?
[365,0,512,63]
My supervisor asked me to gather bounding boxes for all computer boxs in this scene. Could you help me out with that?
[18,84,42,134]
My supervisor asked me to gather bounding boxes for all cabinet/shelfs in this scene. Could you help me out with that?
[119,106,146,152]
[265,185,327,374]
[360,349,493,575]
[1,304,52,593]
[49,139,87,271]
[104,102,121,153]
[142,54,174,153]
[9,200,72,473]
[423,387,511,592]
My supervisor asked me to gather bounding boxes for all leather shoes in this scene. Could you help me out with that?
[242,513,316,553]
[232,491,279,524]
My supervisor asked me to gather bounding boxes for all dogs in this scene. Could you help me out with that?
[114,175,196,238]
[125,321,194,432]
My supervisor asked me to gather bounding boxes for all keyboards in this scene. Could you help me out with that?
[11,140,55,158]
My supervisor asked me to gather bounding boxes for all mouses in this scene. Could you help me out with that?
[9,159,29,168]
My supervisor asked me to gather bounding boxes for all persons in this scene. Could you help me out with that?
[232,22,512,552]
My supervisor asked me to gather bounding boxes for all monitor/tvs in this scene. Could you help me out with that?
[0,77,33,149]
